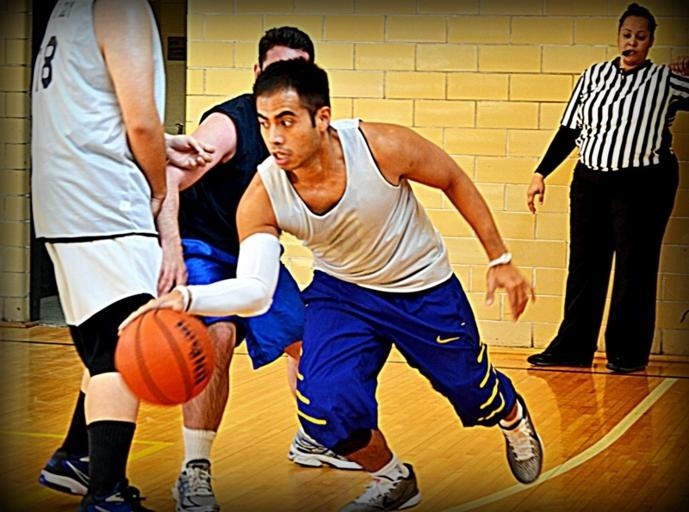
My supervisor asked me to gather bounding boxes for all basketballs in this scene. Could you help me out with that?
[116,310,216,408]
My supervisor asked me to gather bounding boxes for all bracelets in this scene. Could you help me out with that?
[489,253,512,268]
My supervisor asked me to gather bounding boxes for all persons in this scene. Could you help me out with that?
[116,57,543,512]
[526,2,689,372]
[31,0,216,512]
[156,26,365,512]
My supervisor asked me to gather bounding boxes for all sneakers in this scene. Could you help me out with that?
[39,447,156,512]
[338,463,421,512]
[288,426,364,470]
[497,394,544,484]
[170,458,222,512]
[527,351,645,373]
